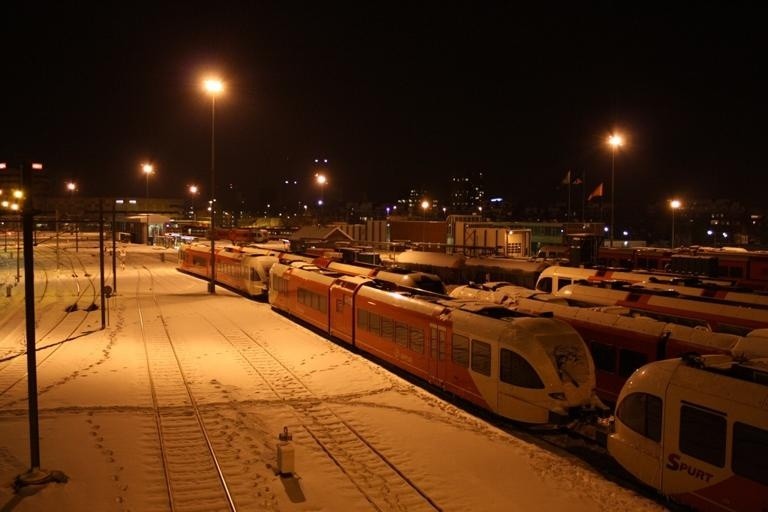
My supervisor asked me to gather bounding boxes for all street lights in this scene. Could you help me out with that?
[190,187,196,220]
[205,79,224,292]
[144,165,151,237]
[422,202,428,241]
[68,183,75,235]
[671,201,680,248]
[610,136,621,247]
[318,176,324,212]
[0,189,21,229]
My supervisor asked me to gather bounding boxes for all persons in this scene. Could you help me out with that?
[537,241,546,257]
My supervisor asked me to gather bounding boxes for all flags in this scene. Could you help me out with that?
[587,183,602,201]
[563,171,570,184]
[573,177,585,185]
